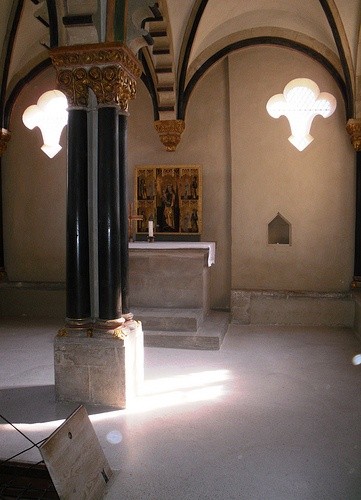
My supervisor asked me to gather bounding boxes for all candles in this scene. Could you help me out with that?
[147,221,153,236]
